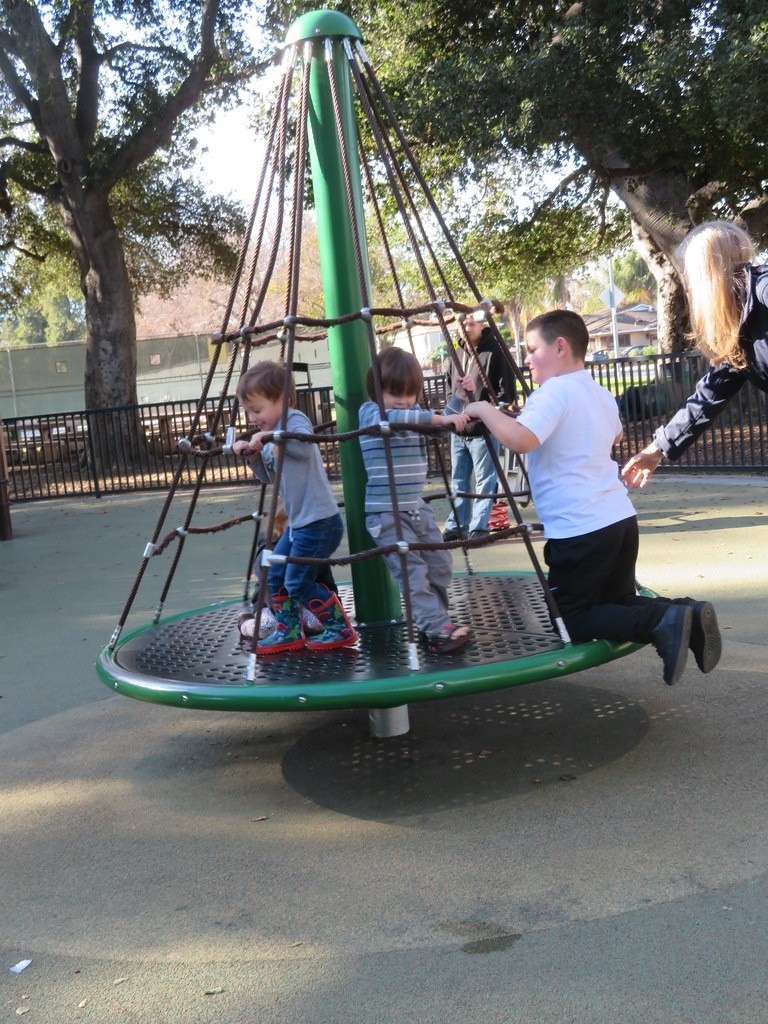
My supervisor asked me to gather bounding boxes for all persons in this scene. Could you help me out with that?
[461,308,725,690]
[354,347,477,652]
[436,308,517,546]
[616,219,767,491]
[226,359,357,656]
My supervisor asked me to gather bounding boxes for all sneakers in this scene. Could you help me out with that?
[443,529,466,540]
[687,601,722,673]
[471,529,489,539]
[654,603,691,686]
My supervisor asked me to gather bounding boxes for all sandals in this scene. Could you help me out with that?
[420,619,469,652]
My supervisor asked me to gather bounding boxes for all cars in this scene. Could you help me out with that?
[584,344,648,362]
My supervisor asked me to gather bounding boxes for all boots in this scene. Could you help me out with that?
[304,590,357,651]
[256,595,308,655]
